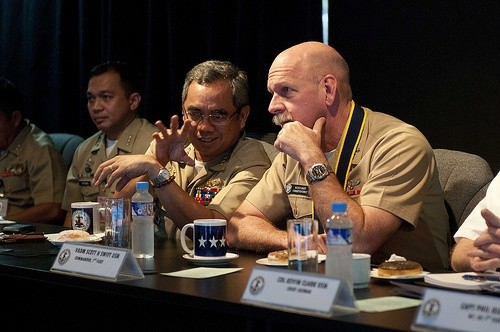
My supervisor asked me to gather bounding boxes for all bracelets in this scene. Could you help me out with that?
[154,175,175,187]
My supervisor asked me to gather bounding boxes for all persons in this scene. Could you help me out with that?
[223,40,451,272]
[449,170,500,272]
[0,75,61,224]
[58,63,162,239]
[90,59,272,241]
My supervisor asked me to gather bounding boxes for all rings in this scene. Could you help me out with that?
[108,165,114,173]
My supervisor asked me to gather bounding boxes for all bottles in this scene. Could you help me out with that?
[131,182,154,258]
[325,202,353,296]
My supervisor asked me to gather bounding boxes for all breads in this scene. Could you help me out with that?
[378,259,422,277]
[268,250,308,262]
[57,230,89,240]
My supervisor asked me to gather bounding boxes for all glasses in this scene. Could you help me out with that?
[182,104,242,122]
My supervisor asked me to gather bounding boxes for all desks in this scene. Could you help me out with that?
[0,224,424,332]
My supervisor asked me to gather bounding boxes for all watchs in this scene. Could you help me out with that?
[150,167,171,187]
[305,161,334,185]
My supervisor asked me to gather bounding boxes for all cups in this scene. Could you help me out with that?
[105,198,131,249]
[71,202,112,234]
[352,253,371,289]
[181,219,227,259]
[287,220,318,272]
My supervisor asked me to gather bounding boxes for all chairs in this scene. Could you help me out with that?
[46,131,84,163]
[427,143,491,221]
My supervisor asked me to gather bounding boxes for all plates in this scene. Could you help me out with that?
[44,234,103,247]
[183,253,239,264]
[370,269,430,279]
[256,258,289,266]
